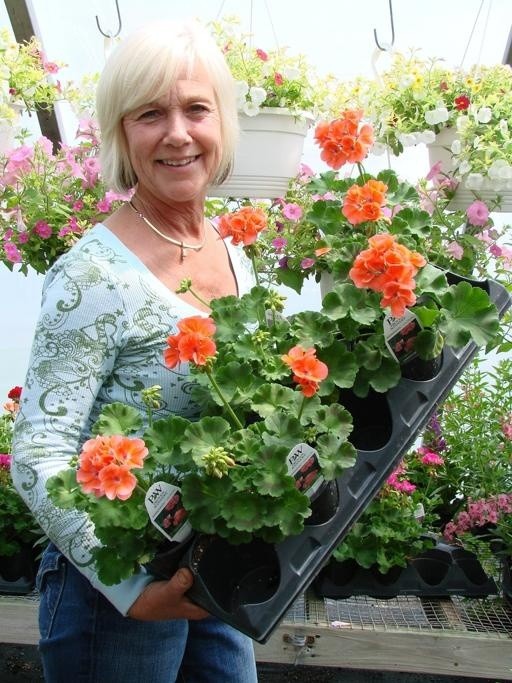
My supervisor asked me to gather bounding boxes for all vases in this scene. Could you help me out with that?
[316,266,343,293]
[430,127,510,186]
[164,278,508,600]
[234,104,313,177]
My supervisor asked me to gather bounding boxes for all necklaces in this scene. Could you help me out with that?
[127,197,207,255]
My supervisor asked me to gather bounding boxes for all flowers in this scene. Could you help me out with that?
[214,23,313,100]
[394,58,508,125]
[80,116,433,522]
[0,31,126,276]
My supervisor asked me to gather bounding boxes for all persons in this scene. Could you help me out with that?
[7,18,262,680]
